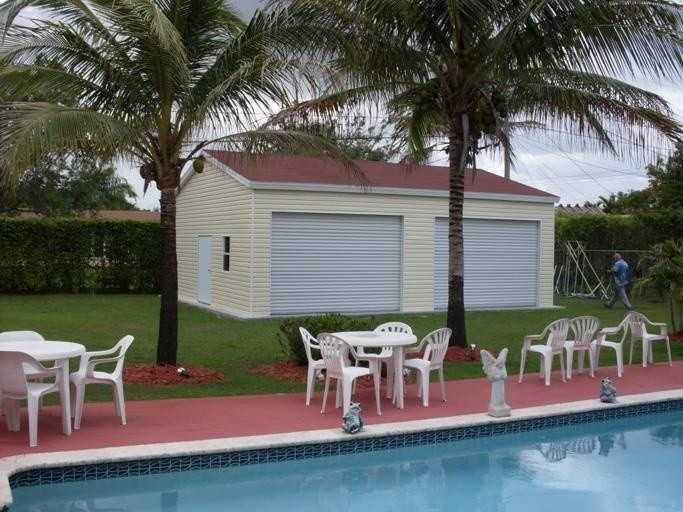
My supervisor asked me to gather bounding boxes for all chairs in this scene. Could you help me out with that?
[589,314,630,379]
[394,328,453,406]
[357,322,413,399]
[518,317,569,386]
[0,330,45,431]
[563,315,600,379]
[0,351,63,447]
[628,311,673,368]
[69,335,134,430]
[298,326,357,409]
[317,333,382,418]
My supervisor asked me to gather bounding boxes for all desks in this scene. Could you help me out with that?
[330,331,417,414]
[0,340,86,436]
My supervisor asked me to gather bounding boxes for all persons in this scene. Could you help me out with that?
[602,253,635,311]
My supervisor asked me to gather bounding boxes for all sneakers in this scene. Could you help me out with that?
[603,303,611,308]
